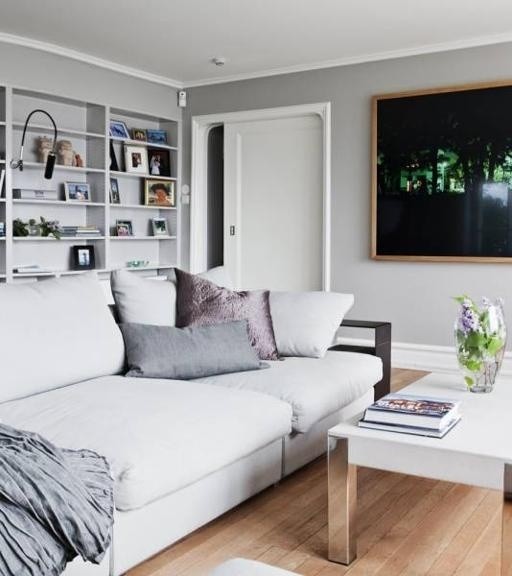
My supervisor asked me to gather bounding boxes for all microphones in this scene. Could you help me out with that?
[44,152,56,179]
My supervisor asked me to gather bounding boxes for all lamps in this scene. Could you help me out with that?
[9,108,58,179]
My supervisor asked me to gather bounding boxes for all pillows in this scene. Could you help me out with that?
[115,319,272,378]
[265,285,358,359]
[111,262,234,327]
[166,266,286,362]
[1,268,126,403]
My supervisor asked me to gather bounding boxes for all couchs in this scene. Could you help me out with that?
[1,275,392,576]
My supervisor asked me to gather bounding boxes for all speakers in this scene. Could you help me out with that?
[179,91,186,107]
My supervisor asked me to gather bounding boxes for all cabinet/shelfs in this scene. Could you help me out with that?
[108,104,182,277]
[0,82,106,283]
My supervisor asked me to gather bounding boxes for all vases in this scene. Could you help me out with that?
[451,288,510,394]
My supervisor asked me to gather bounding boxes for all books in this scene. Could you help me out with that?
[1,222,6,236]
[13,188,57,200]
[0,169,6,197]
[17,268,53,274]
[363,391,459,430]
[14,259,38,268]
[61,224,102,237]
[359,419,463,439]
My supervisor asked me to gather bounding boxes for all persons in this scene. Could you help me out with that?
[75,190,85,199]
[111,123,171,236]
[82,254,89,263]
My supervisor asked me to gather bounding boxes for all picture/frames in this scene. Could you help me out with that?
[62,179,91,203]
[70,243,95,269]
[367,77,511,265]
[109,118,177,239]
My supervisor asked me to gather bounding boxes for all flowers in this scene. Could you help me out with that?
[454,296,505,389]
